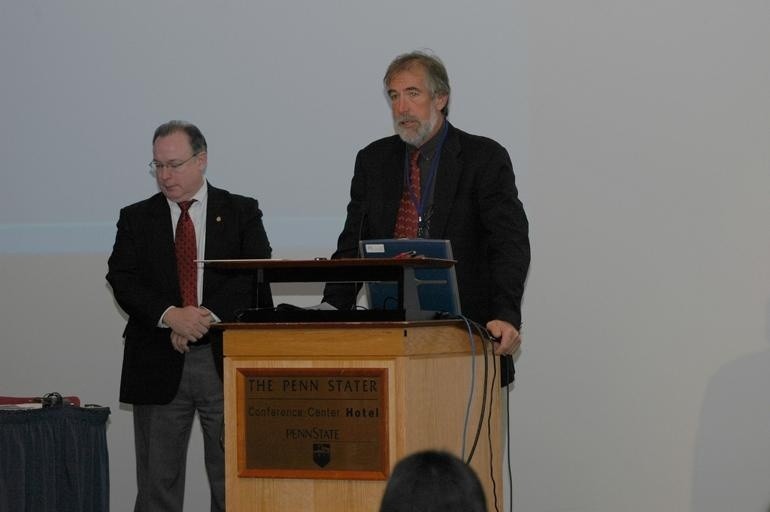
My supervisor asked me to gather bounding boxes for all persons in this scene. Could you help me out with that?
[105,120,272,511]
[309,49,531,389]
[377,447,486,512]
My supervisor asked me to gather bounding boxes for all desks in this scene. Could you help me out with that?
[1,403,112,512]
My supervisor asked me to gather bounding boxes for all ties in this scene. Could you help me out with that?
[174,199,198,310]
[392,148,423,240]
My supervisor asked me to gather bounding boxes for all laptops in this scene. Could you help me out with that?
[359,238,496,340]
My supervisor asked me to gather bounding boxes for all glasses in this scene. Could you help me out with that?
[148,154,197,172]
